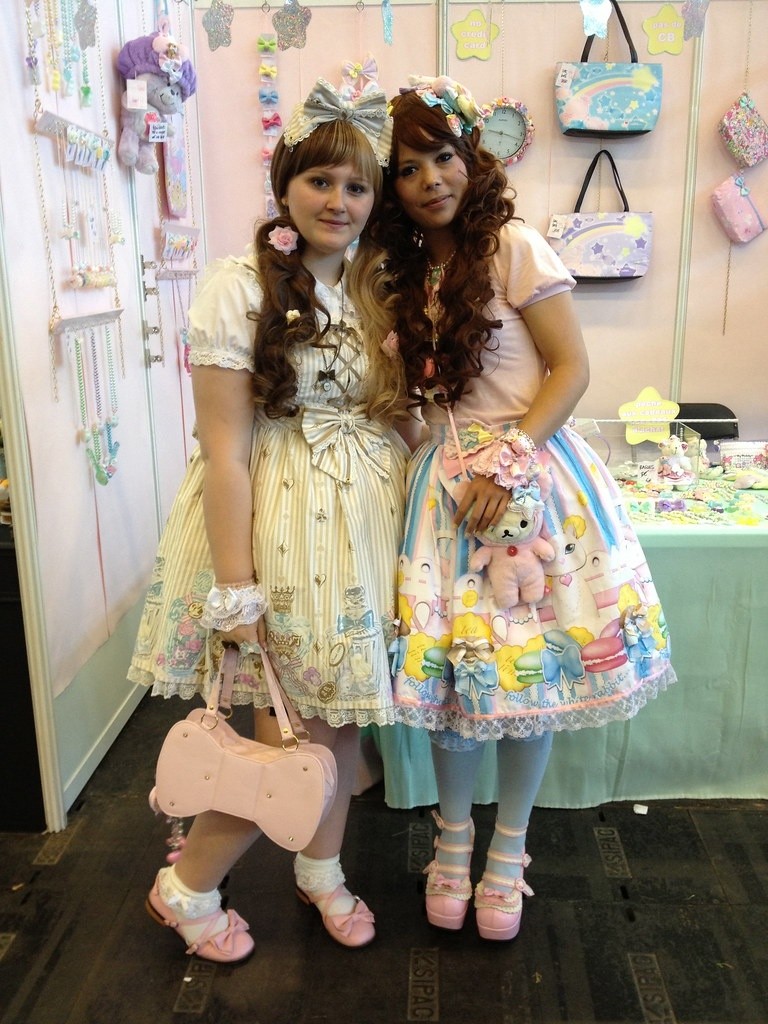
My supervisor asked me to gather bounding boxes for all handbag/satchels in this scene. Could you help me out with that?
[148,643,338,853]
[710,171,767,245]
[555,0,664,139]
[669,403,739,441]
[717,89,768,169]
[547,150,654,284]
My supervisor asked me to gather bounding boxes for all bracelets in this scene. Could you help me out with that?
[200,572,270,633]
[468,431,545,490]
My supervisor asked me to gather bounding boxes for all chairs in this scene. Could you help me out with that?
[630,402,740,463]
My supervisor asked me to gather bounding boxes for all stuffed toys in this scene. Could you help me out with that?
[457,459,555,609]
[117,26,198,173]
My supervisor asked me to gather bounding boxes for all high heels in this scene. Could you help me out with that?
[473,814,536,942]
[422,809,476,931]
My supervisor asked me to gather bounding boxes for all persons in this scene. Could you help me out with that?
[346,77,679,945]
[124,93,434,964]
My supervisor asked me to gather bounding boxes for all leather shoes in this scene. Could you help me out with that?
[296,879,377,950]
[144,873,255,966]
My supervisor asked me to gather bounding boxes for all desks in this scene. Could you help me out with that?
[373,456,768,809]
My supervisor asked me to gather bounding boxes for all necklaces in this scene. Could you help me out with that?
[419,237,460,274]
